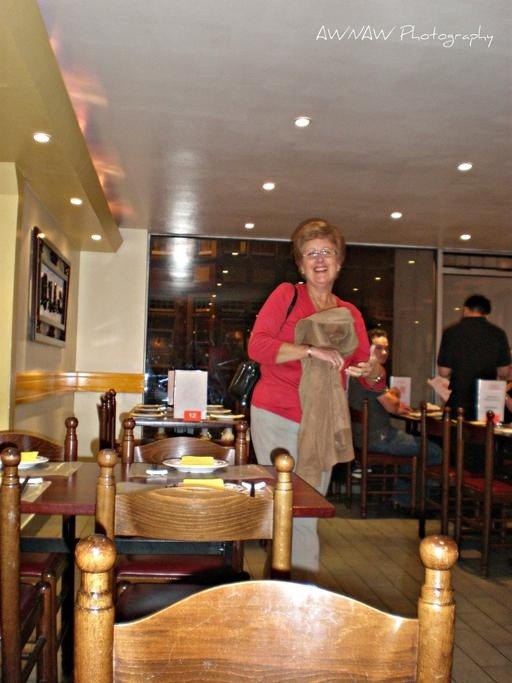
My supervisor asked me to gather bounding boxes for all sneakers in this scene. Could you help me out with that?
[424,496,445,513]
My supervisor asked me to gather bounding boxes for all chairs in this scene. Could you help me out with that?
[0,362,512,682]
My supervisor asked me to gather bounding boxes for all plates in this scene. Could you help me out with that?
[161,481,247,492]
[133,403,165,420]
[206,404,245,419]
[163,457,230,472]
[17,454,51,471]
[409,412,443,418]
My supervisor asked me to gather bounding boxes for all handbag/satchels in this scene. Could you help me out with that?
[229,359,262,401]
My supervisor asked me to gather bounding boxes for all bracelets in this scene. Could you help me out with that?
[308,344,313,360]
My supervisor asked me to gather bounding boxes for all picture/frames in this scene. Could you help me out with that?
[27,222,74,350]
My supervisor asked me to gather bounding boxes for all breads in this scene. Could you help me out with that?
[183,478,224,492]
[181,455,214,467]
[18,451,39,463]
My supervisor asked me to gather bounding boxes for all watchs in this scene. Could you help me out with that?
[369,370,385,384]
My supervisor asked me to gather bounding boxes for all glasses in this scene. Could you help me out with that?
[302,248,338,257]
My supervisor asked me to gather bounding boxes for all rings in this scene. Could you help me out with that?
[364,372,367,376]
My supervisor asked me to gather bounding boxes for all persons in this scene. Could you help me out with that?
[346,330,444,511]
[437,295,511,421]
[248,217,386,574]
